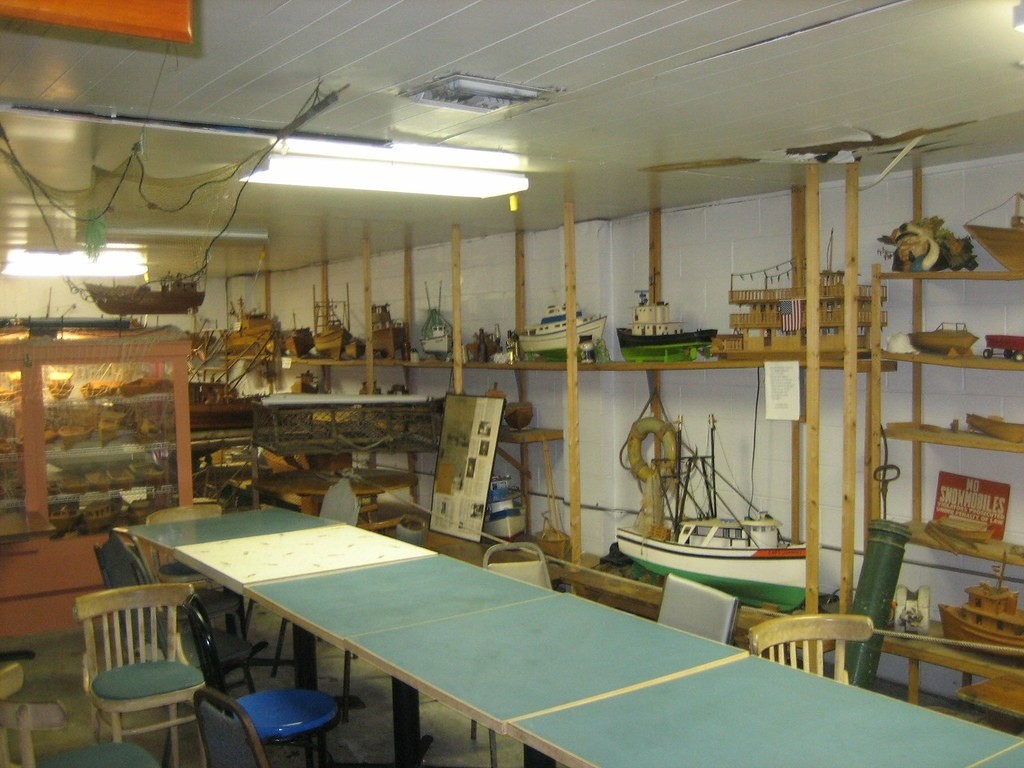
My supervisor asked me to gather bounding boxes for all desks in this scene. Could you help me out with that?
[109,464,1024,768]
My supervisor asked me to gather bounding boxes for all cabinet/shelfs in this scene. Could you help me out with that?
[0,161,1024,706]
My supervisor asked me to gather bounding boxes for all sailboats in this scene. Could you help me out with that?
[535,434,570,559]
[615,413,823,615]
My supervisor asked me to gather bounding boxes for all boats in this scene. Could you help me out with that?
[963,192,1024,272]
[907,321,979,355]
[937,548,1024,668]
[965,412,1024,444]
[500,401,536,432]
[482,474,527,544]
[711,228,889,362]
[420,324,453,356]
[616,301,719,363]
[372,302,405,359]
[1,371,267,534]
[519,302,608,363]
[223,298,365,361]
[82,271,206,316]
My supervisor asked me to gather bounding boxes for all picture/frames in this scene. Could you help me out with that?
[422,394,506,543]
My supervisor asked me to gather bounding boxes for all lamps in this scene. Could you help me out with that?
[1,249,148,278]
[1010,0,1024,34]
[236,132,533,202]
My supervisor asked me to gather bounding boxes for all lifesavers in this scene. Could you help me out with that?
[627,417,679,483]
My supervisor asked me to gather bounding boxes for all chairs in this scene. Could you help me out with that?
[109,525,246,641]
[468,541,554,768]
[747,608,875,686]
[0,699,163,768]
[145,501,225,592]
[192,685,274,768]
[180,590,344,768]
[93,534,266,700]
[654,571,742,645]
[73,578,208,768]
